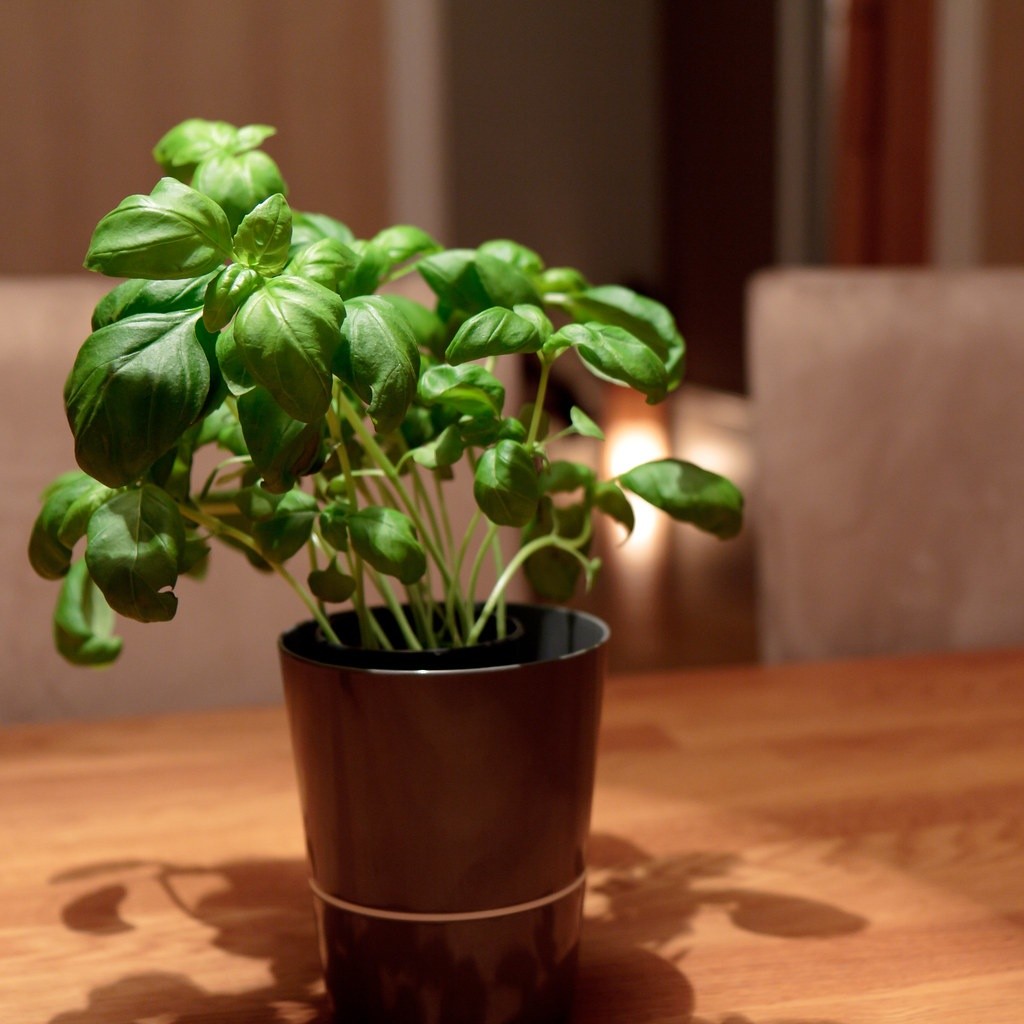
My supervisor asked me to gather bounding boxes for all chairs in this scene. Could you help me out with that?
[0,276,525,727]
[748,266,1023,656]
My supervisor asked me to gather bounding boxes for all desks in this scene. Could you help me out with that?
[1,644,1023,1024]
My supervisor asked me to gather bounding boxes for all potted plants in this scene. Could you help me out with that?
[29,119,748,1022]
